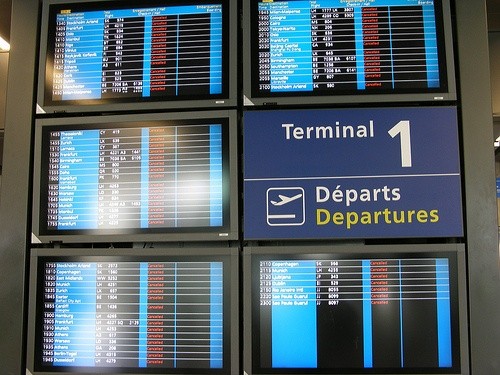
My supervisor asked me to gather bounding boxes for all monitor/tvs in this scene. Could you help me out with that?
[35,0,469,374]
